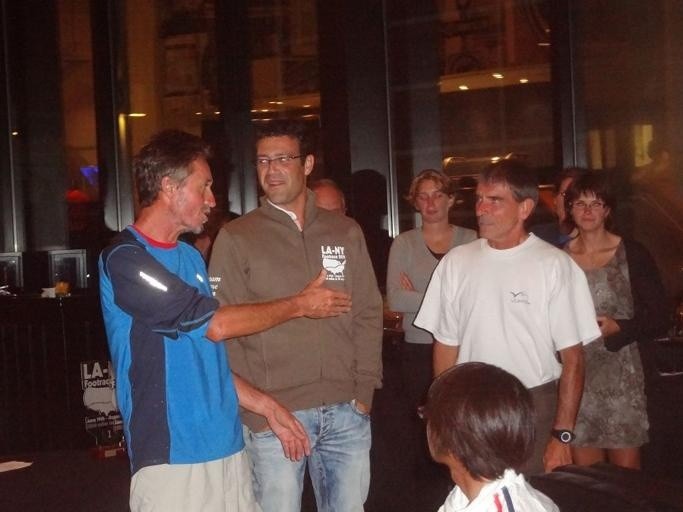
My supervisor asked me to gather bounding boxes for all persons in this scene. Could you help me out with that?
[387,160,669,511]
[98,120,384,512]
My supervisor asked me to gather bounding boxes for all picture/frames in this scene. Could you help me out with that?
[1,251,25,293]
[47,247,88,292]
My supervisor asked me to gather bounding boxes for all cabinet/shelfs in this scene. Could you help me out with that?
[1,284,123,459]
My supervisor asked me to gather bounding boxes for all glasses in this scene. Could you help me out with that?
[568,200,607,209]
[252,154,305,168]
[417,406,429,420]
